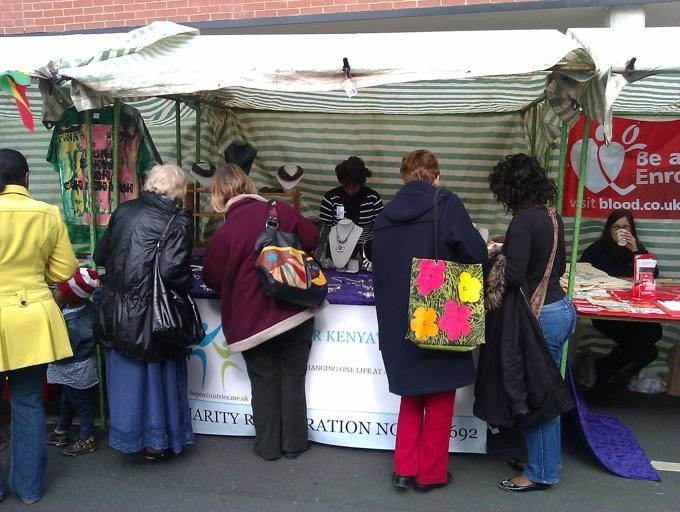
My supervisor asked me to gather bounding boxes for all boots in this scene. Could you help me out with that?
[591,346,639,410]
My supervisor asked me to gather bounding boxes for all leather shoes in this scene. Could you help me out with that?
[509,458,528,471]
[498,475,551,491]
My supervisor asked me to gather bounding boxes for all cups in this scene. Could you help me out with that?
[616,228,629,247]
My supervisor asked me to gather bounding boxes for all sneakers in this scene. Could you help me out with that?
[414,472,453,491]
[44,428,68,447]
[62,436,97,457]
[146,447,181,462]
[393,471,410,488]
[282,441,312,460]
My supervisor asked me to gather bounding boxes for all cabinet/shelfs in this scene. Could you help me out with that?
[185,183,302,232]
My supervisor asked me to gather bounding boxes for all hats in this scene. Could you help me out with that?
[58,266,100,303]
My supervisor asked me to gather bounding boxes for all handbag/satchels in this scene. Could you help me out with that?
[253,200,329,307]
[148,253,206,352]
[403,257,487,352]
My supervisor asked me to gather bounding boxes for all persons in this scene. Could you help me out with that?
[489,153,577,493]
[90,161,203,463]
[578,208,664,405]
[200,162,322,462]
[0,146,78,506]
[45,264,102,459]
[316,156,385,259]
[368,146,491,492]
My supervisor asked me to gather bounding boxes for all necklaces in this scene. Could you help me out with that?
[336,224,355,252]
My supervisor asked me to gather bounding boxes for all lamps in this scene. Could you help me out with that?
[338,55,358,101]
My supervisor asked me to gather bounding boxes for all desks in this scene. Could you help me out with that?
[560,277,680,384]
[184,260,488,454]
